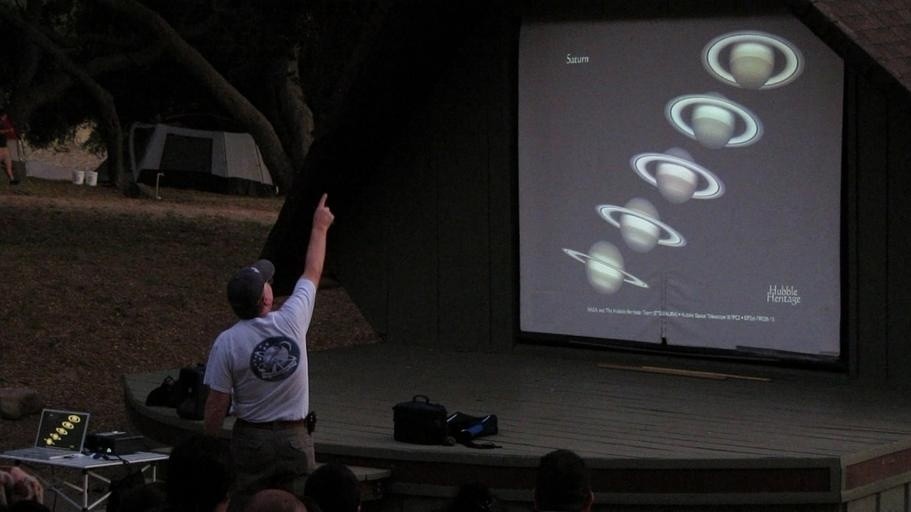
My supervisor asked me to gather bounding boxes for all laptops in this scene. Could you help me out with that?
[4,408,90,460]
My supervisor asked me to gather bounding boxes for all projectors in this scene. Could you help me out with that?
[86,432,145,455]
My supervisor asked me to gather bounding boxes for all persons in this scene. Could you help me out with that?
[0,111,20,186]
[203,193,335,501]
[0,449,598,511]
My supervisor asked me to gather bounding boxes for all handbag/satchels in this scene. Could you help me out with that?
[392,394,450,442]
[146,376,175,408]
[175,365,232,421]
[448,411,499,435]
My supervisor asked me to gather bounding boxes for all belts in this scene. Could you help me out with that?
[240,419,305,430]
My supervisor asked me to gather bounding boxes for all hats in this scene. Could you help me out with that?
[227,259,275,317]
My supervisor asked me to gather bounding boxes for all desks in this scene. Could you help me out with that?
[0,447,170,512]
